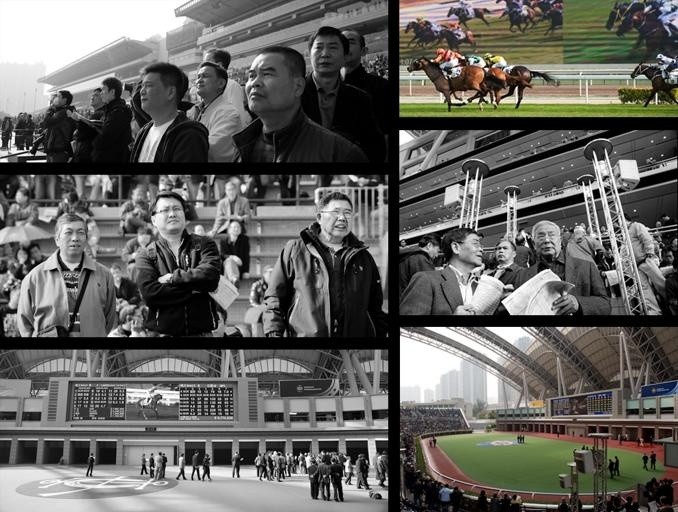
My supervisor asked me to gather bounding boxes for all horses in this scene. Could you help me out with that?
[604,0,678,63]
[405,0,564,53]
[407,55,556,112]
[630,61,678,107]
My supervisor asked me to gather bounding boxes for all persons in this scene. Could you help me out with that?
[400,408,675,511]
[0,20,387,163]
[86,452,96,477]
[416,48,678,80]
[145,386,157,403]
[417,0,563,39]
[657,0,678,38]
[191,451,201,480]
[201,454,211,481]
[253,450,388,502]
[231,451,244,478]
[140,451,167,480]
[175,454,187,480]
[400,210,678,315]
[1,175,388,339]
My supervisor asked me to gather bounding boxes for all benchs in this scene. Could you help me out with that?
[0,203,317,308]
